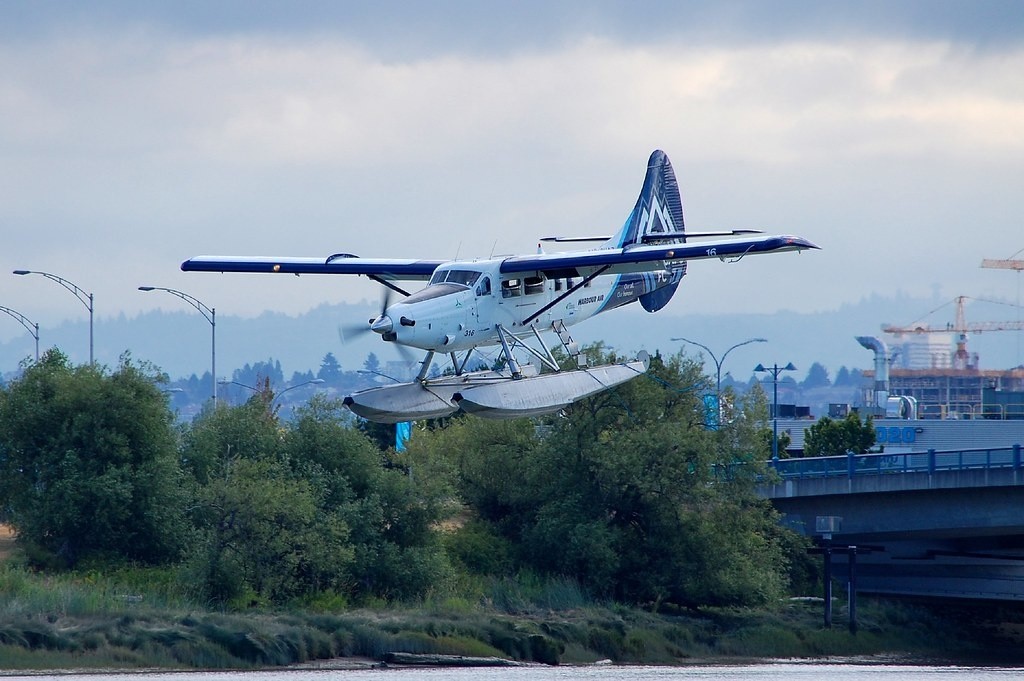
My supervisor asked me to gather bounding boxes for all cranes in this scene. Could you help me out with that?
[881,294,1024,350]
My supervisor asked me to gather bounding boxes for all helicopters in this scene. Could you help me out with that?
[181,150,823,425]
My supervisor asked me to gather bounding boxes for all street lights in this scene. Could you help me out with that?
[139,286,216,412]
[666,337,768,425]
[753,361,798,461]
[12,269,93,370]
